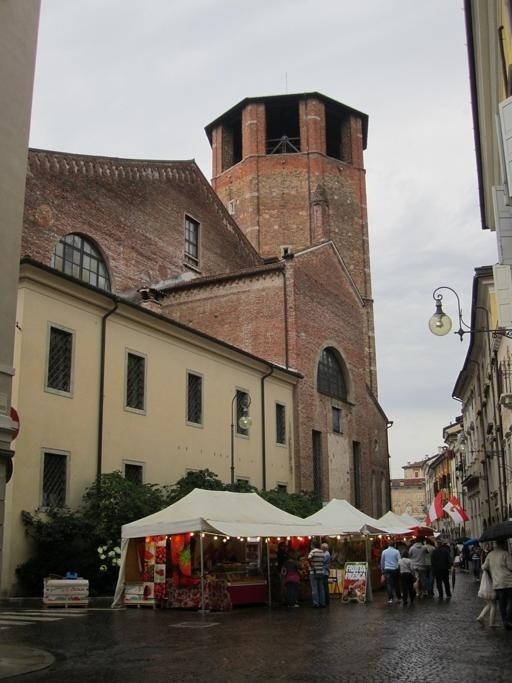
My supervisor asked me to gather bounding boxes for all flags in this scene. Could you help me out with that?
[441,495,470,523]
[424,490,446,526]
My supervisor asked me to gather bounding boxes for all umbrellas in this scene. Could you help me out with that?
[479,520,511,541]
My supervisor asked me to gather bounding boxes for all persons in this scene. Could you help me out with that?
[308,540,326,608]
[250,556,284,604]
[480,538,511,630]
[277,541,290,568]
[321,542,331,605]
[476,547,501,628]
[283,559,300,608]
[371,534,481,606]
[480,542,489,562]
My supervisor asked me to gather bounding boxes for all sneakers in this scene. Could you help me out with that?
[476,616,502,629]
[386,598,402,604]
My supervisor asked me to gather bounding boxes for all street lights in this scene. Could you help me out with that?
[429,286,512,342]
[231,392,254,489]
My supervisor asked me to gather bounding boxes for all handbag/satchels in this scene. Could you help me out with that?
[380,575,386,584]
